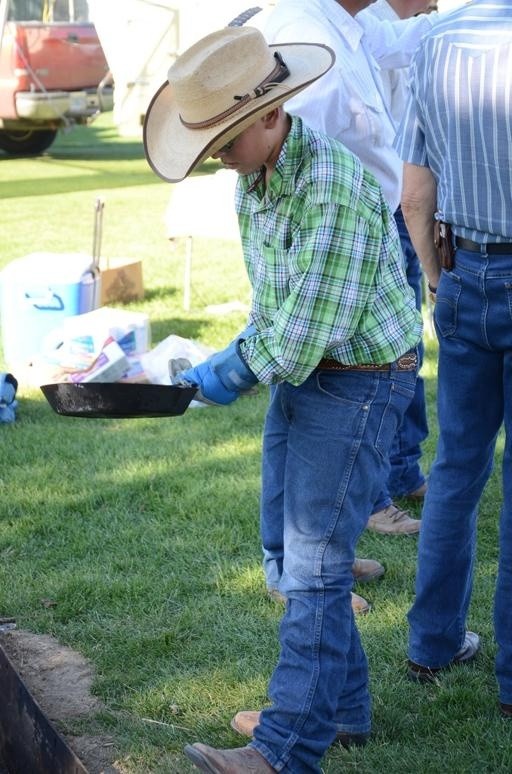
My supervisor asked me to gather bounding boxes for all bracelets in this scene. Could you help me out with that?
[427,283,437,294]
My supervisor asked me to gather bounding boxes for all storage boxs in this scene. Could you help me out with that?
[0,250,104,322]
[97,252,145,312]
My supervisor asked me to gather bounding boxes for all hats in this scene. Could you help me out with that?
[142,26,337,183]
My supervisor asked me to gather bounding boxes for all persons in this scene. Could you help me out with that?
[387,0,512,717]
[350,1,440,540]
[171,103,427,774]
[237,0,405,620]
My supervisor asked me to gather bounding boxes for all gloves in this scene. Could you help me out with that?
[167,337,257,407]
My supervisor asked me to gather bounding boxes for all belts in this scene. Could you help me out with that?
[456,238,512,254]
[317,354,419,371]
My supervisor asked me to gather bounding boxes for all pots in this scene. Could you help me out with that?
[39,381,201,420]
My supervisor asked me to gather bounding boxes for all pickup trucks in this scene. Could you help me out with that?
[0,0,114,156]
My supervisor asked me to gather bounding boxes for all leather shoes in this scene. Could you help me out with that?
[184,743,276,773]
[350,558,385,578]
[367,507,420,534]
[408,631,479,680]
[351,591,368,612]
[231,710,367,752]
